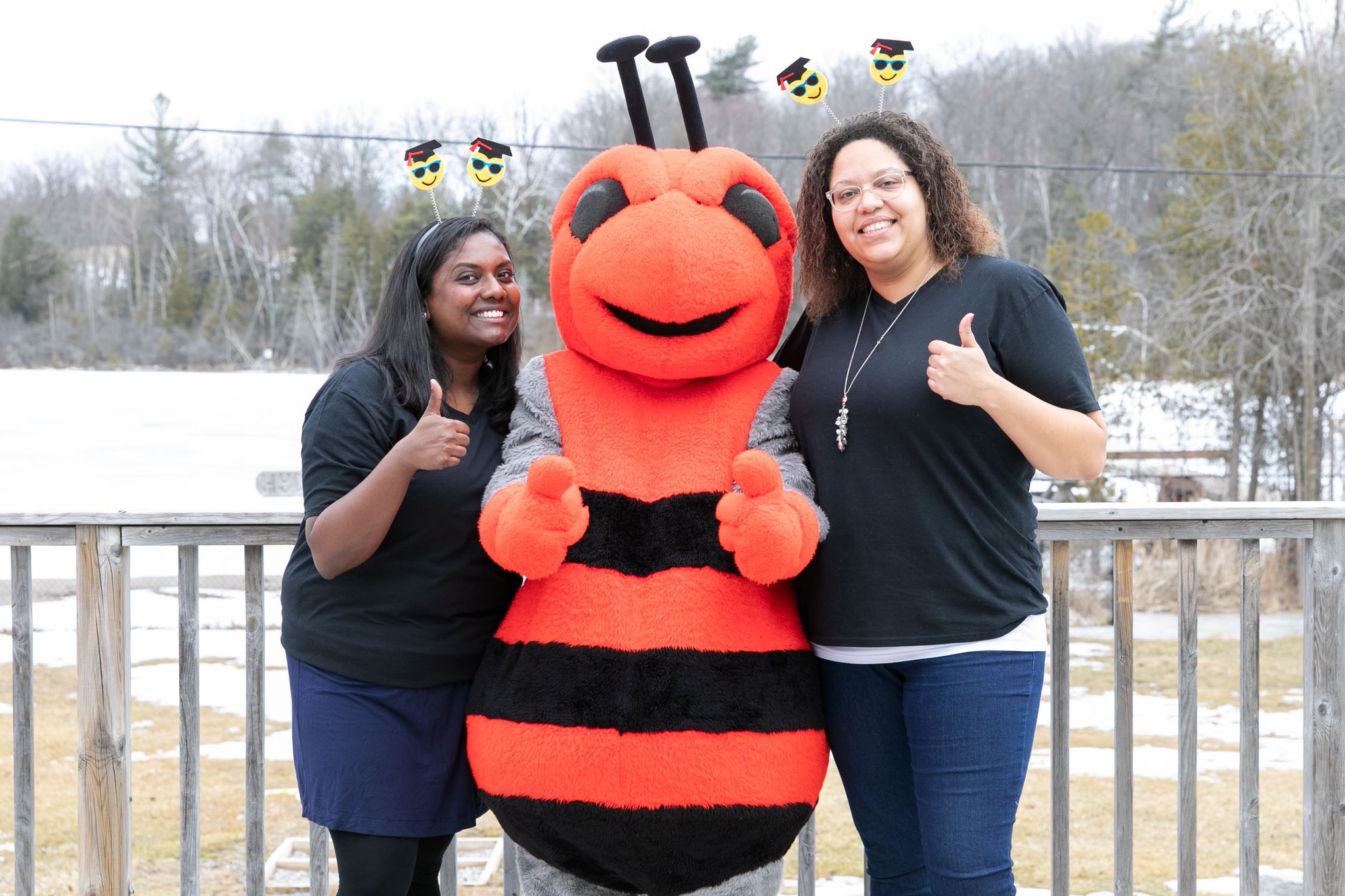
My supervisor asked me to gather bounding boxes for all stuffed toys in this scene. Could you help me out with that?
[465,37,829,896]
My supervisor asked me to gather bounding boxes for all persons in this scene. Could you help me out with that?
[773,111,1109,896]
[281,217,525,896]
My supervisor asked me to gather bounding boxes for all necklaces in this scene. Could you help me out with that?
[835,256,938,452]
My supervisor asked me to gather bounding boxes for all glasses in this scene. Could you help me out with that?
[825,169,920,211]
